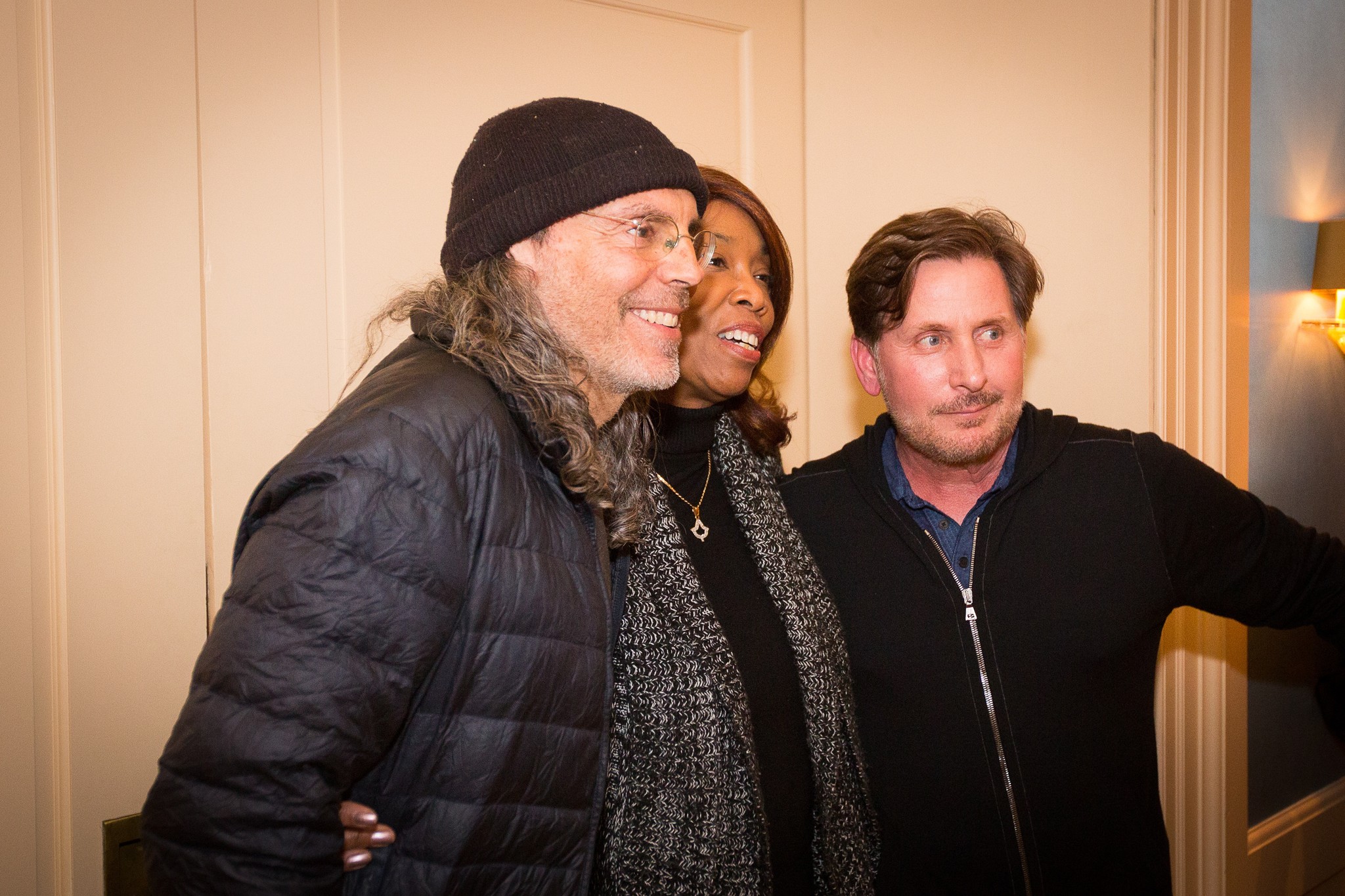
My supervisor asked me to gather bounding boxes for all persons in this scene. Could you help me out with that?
[142,98,707,896]
[339,167,888,896]
[772,208,1345,896]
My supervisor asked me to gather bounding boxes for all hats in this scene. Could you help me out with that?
[440,97,710,284]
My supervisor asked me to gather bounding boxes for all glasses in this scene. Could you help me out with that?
[580,210,717,268]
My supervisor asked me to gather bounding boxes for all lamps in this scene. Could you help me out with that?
[1303,221,1344,354]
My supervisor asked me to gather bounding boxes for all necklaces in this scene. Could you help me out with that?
[656,448,712,542]
